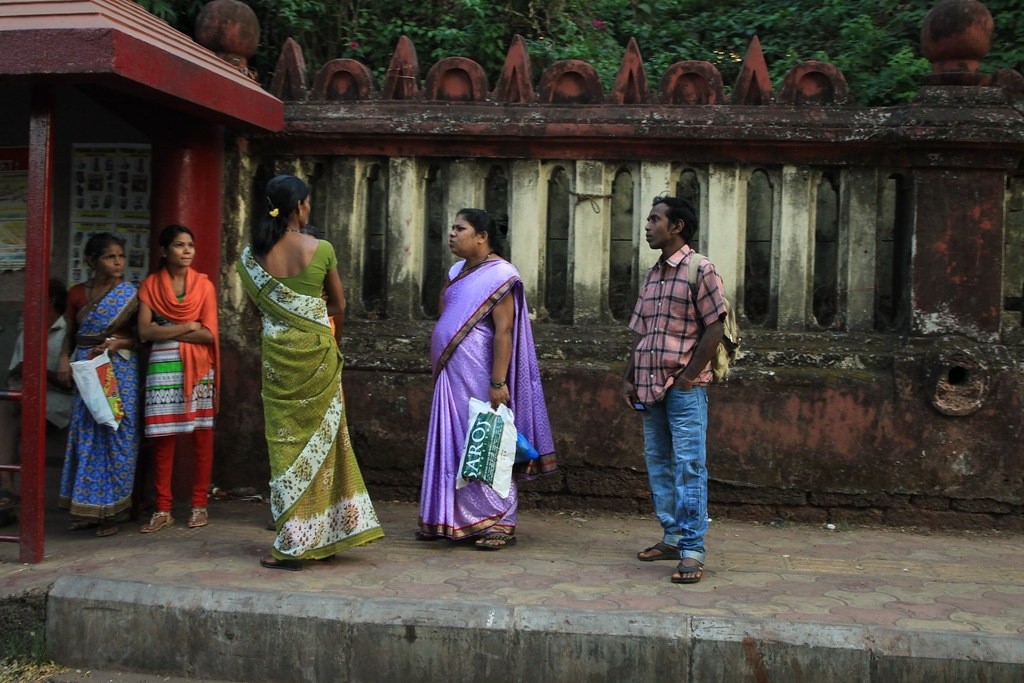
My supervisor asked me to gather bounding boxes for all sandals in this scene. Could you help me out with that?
[140,511,175,533]
[187,508,208,528]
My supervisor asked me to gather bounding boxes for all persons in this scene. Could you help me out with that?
[0,224,219,532]
[415,208,558,550]
[236,174,384,571]
[622,196,727,583]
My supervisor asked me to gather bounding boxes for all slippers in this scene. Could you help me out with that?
[671,562,703,583]
[474,534,516,549]
[415,531,444,540]
[638,543,680,561]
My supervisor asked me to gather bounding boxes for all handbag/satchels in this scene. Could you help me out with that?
[515,430,538,463]
[456,397,518,499]
[69,348,124,432]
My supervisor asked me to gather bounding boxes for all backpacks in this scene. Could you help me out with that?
[688,254,739,384]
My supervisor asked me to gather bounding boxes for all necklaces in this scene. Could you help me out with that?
[286,230,297,232]
[89,277,117,307]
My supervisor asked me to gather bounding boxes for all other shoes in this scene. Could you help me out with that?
[95,522,122,537]
[0,491,18,512]
[260,554,303,571]
[67,521,96,531]
[0,513,17,528]
[322,554,336,562]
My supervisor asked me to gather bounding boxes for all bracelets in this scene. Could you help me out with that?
[680,375,691,382]
[490,381,506,387]
